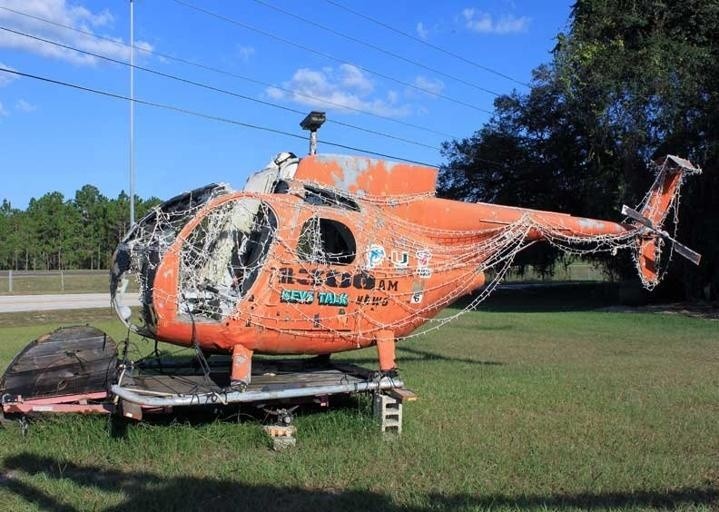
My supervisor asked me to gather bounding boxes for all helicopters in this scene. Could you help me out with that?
[109,112,704,388]
